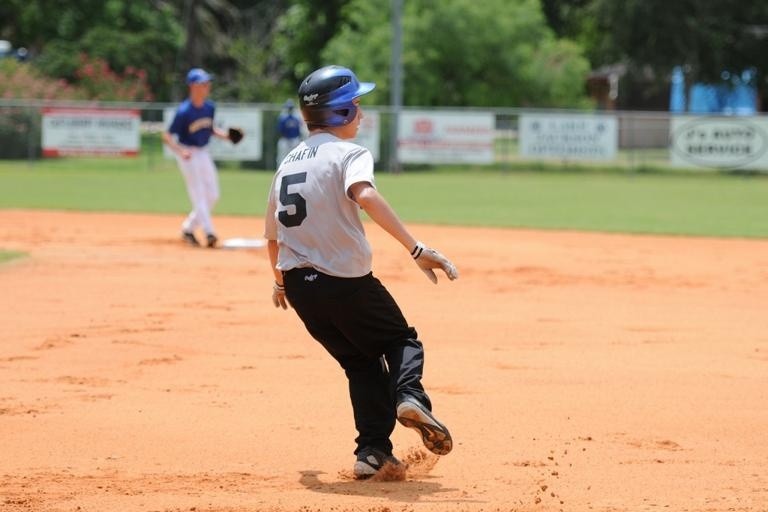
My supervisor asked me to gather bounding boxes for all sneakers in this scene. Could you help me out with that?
[354,448,406,481]
[396,394,453,455]
[181,230,217,246]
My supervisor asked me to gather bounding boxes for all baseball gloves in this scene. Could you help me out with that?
[229,129,243,143]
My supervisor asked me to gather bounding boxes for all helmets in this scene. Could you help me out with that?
[298,63,376,128]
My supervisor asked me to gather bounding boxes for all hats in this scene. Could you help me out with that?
[186,67,215,88]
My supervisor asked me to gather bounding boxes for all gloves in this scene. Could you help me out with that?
[271,281,294,310]
[412,239,459,284]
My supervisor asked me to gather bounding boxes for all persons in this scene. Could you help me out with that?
[161,68,244,248]
[275,98,306,171]
[263,65,460,476]
